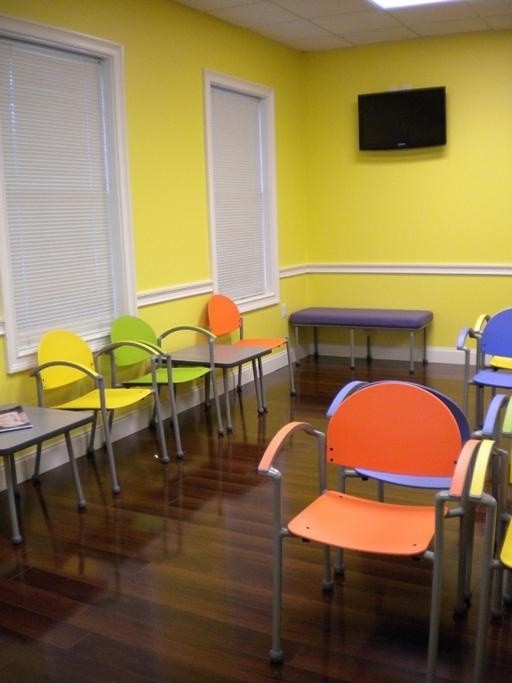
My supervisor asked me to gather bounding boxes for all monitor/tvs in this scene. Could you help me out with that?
[358,87,446,151]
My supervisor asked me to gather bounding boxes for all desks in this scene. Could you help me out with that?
[2,404,97,544]
[287,306,433,396]
[150,342,272,434]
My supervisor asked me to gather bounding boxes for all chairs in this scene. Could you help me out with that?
[207,295,287,418]
[453,293,512,683]
[258,380,474,680]
[108,315,226,455]
[34,329,170,498]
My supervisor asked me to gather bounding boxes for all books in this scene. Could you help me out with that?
[0,403,33,434]
